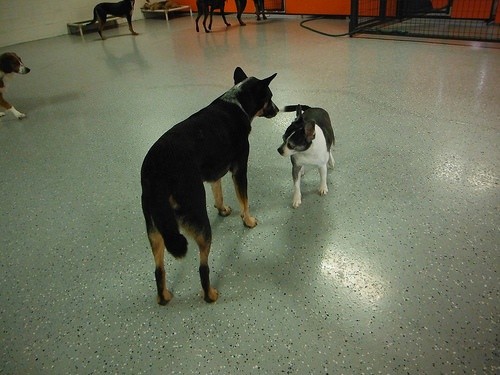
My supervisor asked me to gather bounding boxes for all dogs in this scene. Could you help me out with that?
[141,66,279,304]
[277,104,336,209]
[0,51,30,119]
[85,0,140,40]
[140,0,182,15]
[236,0,267,26]
[195,0,231,33]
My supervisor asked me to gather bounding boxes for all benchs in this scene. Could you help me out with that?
[142,5,193,22]
[67,16,121,36]
